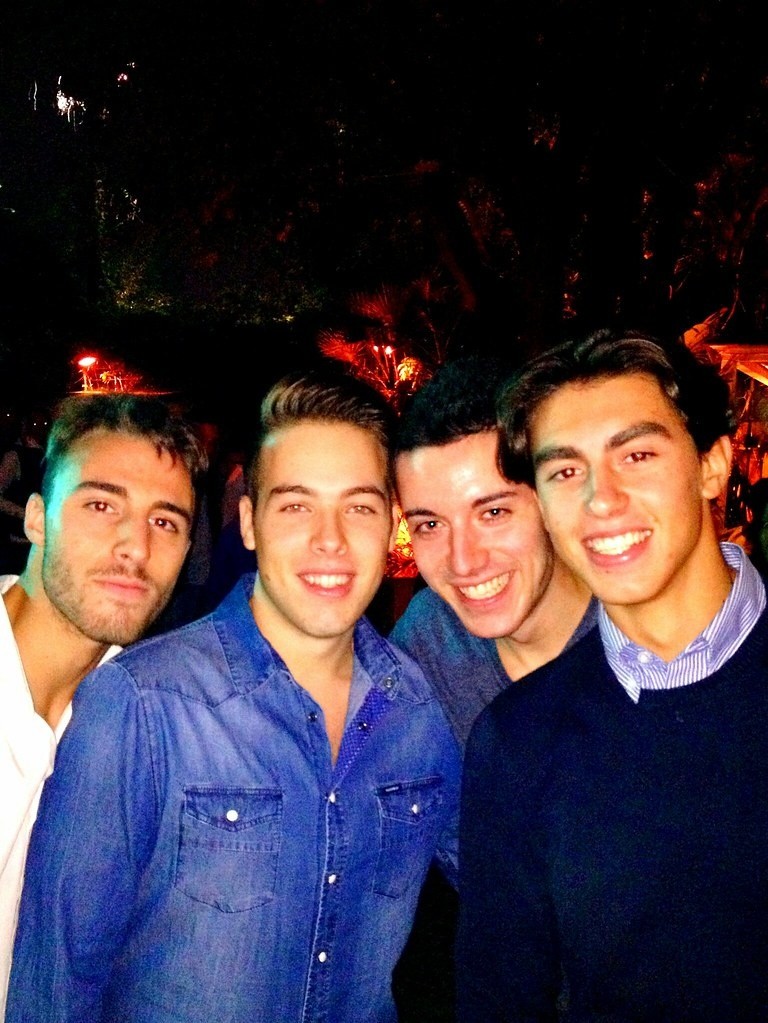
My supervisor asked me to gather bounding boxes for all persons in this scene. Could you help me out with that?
[0,333,768,1023]
